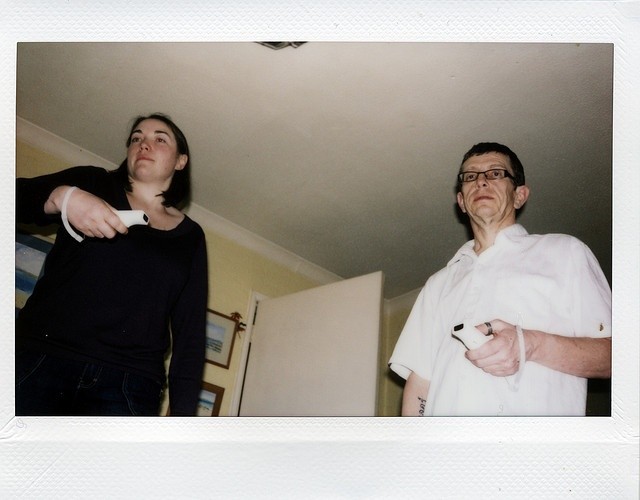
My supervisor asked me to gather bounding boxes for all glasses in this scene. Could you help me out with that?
[457,169,517,182]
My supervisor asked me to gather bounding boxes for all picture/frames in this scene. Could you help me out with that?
[205,307,240,370]
[166,380,226,416]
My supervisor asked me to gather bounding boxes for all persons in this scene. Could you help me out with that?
[17,111,210,415]
[386,138,611,414]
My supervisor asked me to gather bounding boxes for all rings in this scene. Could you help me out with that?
[484,321,493,337]
